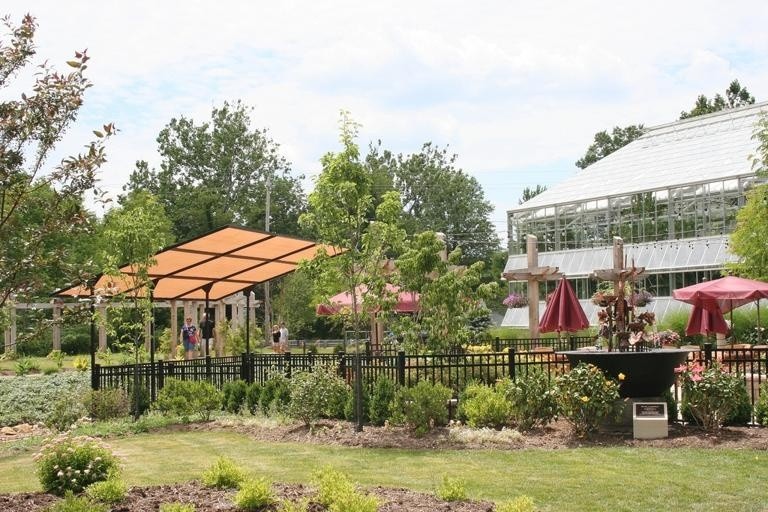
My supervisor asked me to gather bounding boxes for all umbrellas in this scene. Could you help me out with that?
[536,273,591,350]
[315,278,422,348]
[672,275,768,349]
[684,296,731,342]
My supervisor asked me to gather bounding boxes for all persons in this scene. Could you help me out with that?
[278,322,289,353]
[197,312,218,358]
[179,316,202,360]
[271,324,282,354]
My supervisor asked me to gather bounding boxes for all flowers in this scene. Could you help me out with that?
[590,279,660,353]
[502,292,528,309]
